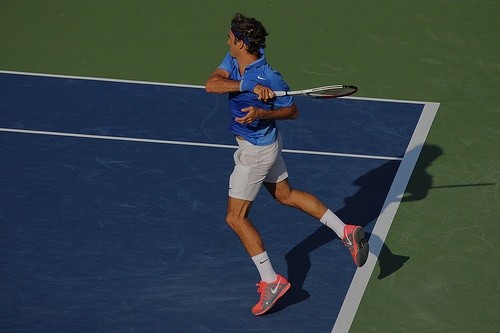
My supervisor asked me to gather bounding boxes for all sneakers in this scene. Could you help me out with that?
[341,224,369,267]
[252,274,291,315]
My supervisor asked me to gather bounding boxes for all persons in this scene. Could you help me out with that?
[204,10,370,317]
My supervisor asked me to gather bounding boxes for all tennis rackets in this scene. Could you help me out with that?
[257,84,358,100]
